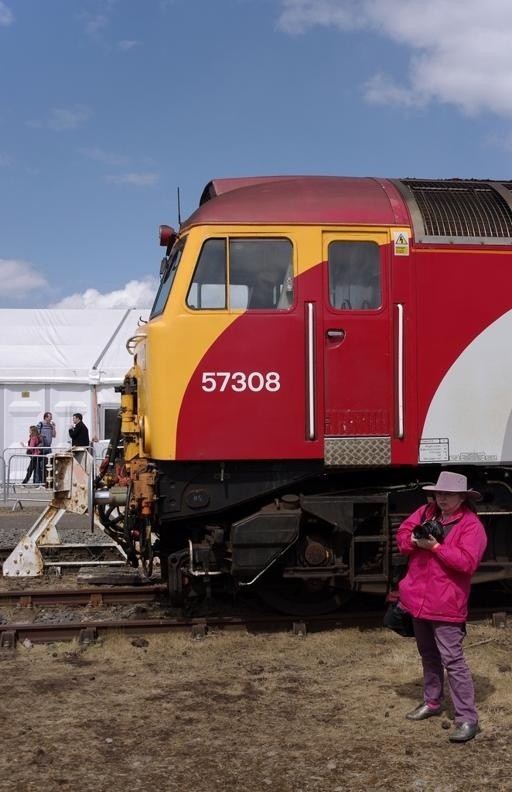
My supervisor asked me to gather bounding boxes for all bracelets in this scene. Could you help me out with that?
[431,543,441,551]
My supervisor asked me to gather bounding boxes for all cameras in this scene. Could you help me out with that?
[413,520,444,542]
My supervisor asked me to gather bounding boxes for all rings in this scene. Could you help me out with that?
[428,540,430,543]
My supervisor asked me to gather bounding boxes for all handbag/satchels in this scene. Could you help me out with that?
[381,595,417,639]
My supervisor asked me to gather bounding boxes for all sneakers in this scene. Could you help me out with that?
[448,723,479,743]
[405,703,444,721]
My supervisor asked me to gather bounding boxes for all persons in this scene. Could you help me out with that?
[36,412,56,483]
[21,425,41,488]
[69,413,89,447]
[396,470,490,743]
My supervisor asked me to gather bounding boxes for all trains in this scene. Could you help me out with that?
[87,175,512,617]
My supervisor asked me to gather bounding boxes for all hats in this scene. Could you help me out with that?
[419,469,480,503]
